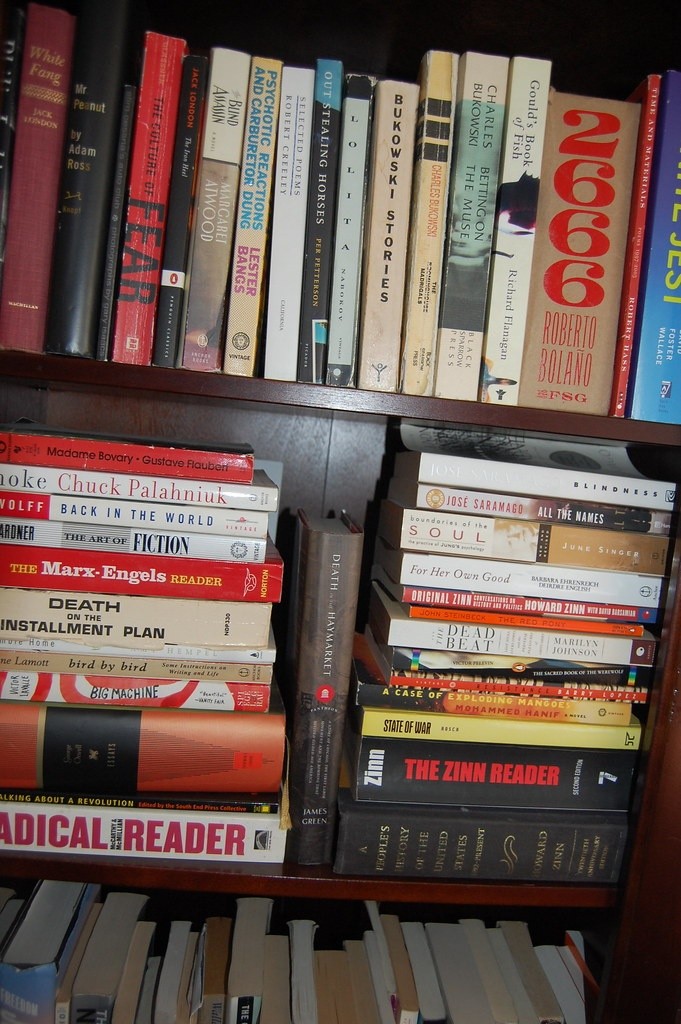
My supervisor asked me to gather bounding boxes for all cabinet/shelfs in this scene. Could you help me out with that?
[0,0,681,1024]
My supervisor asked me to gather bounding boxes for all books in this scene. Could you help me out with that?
[0,880,588,1024]
[336,424,676,882]
[0,1,681,423]
[0,426,363,868]
